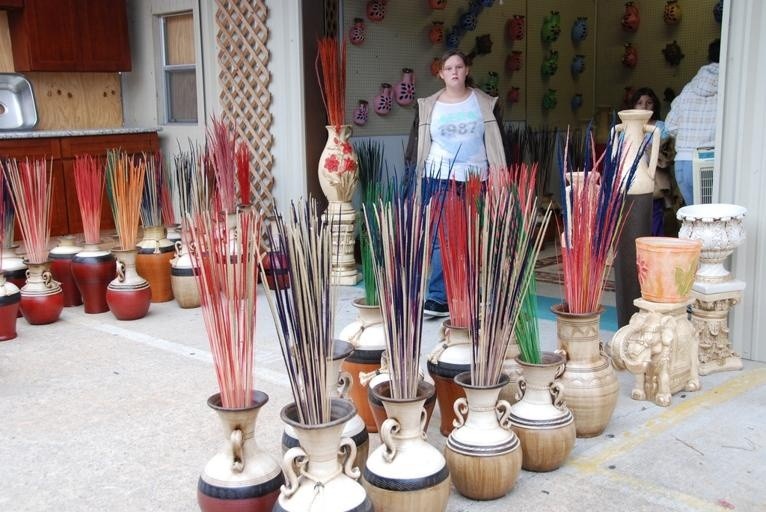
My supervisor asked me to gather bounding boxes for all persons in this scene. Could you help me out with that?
[665,36,722,207]
[414,49,510,317]
[618,87,669,238]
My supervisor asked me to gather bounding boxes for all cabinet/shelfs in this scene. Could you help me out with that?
[1,124,162,241]
[7,1,131,72]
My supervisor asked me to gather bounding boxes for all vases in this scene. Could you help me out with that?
[363,197,451,511]
[445,186,555,503]
[493,165,578,472]
[49,239,84,306]
[3,155,63,326]
[498,332,521,406]
[347,195,385,432]
[104,148,152,321]
[1,271,19,342]
[256,198,375,512]
[1,187,28,320]
[317,122,360,201]
[365,351,437,444]
[284,337,368,481]
[72,154,116,313]
[136,126,293,308]
[550,126,657,436]
[185,210,284,511]
[427,172,486,438]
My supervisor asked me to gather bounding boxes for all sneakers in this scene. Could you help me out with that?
[423,300,450,317]
